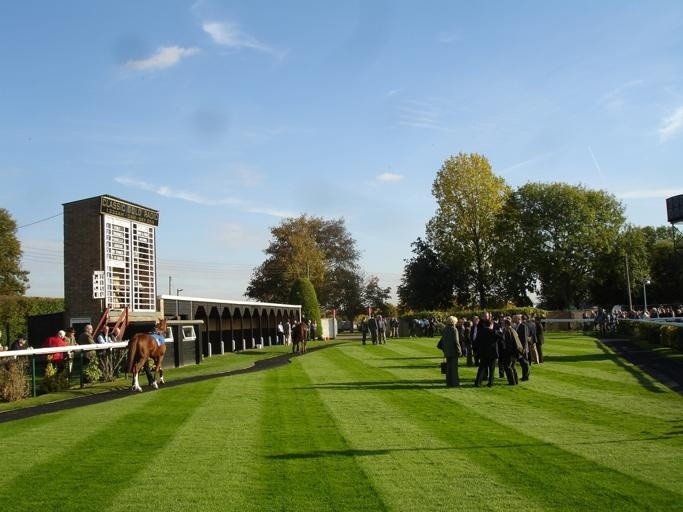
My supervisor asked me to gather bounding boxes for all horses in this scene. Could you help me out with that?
[126,316,168,393]
[292,321,308,354]
[409,317,430,338]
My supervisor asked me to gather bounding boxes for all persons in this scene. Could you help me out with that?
[427,318,435,338]
[107,327,123,378]
[441,315,462,387]
[0,342,8,352]
[76,324,95,367]
[41,329,68,375]
[359,314,399,346]
[9,334,33,351]
[580,305,683,338]
[452,309,544,388]
[94,326,114,382]
[61,326,79,374]
[276,318,317,347]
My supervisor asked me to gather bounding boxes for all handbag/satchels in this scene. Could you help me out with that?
[437,336,444,350]
[441,363,447,374]
